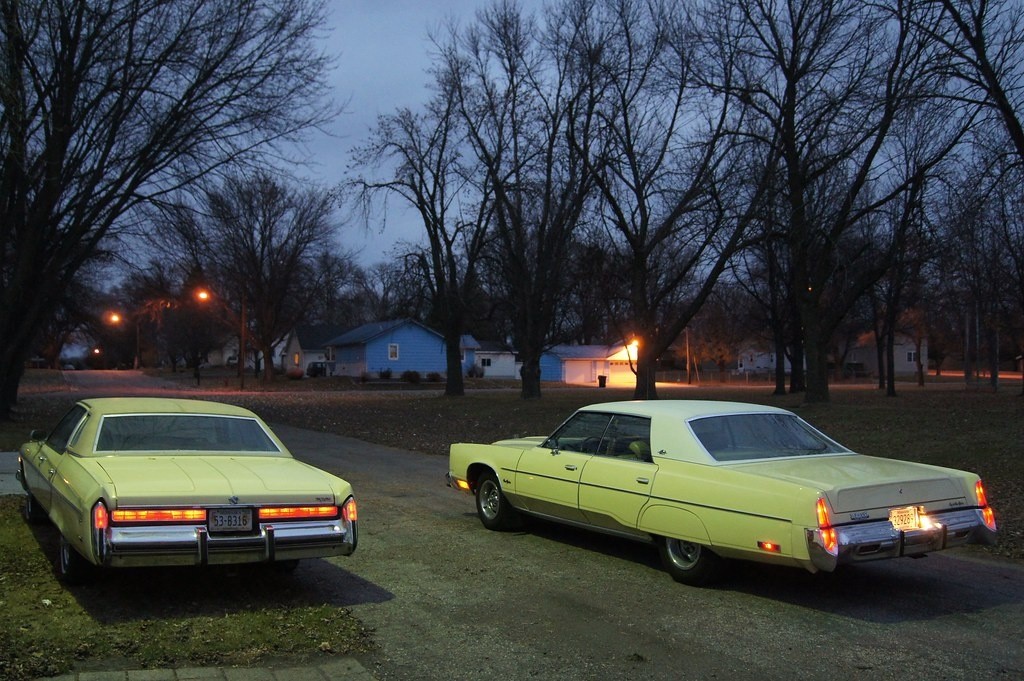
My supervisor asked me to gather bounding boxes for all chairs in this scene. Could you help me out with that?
[630,440,651,462]
[700,430,728,451]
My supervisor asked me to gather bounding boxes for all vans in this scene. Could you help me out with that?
[306,362,326,377]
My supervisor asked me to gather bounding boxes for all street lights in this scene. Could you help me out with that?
[199,290,246,391]
[111,314,139,370]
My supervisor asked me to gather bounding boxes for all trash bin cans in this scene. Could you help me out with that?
[598,375,607,387]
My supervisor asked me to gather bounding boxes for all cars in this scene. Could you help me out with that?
[16,397,358,589]
[446,400,1000,584]
